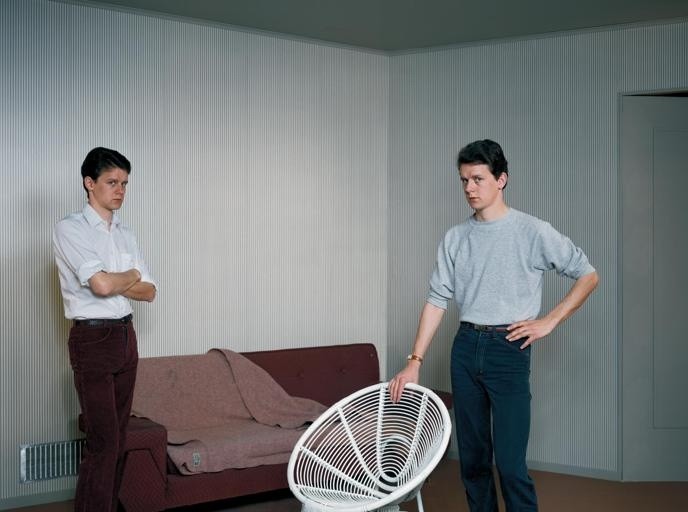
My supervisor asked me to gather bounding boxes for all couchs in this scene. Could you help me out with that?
[77,342,455,511]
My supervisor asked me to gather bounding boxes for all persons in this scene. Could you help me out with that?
[51,147,159,509]
[387,138,600,512]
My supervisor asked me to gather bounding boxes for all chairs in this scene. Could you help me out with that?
[283,379,455,511]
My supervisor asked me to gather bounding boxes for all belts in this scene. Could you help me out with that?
[460,321,507,332]
[75,313,133,326]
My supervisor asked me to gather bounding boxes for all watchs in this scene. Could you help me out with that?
[407,354,424,366]
